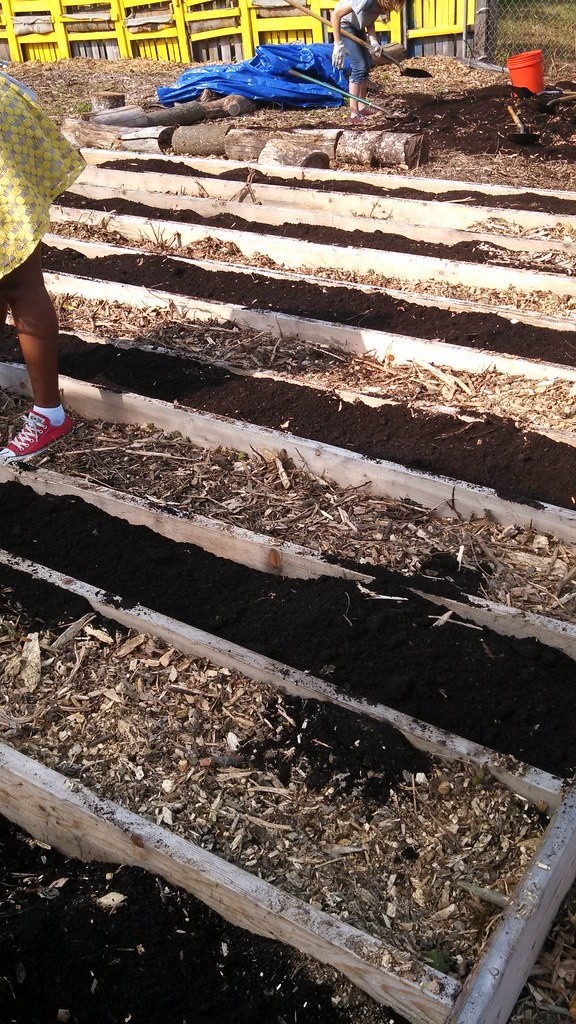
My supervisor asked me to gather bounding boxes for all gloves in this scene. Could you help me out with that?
[369,41,383,60]
[331,40,352,70]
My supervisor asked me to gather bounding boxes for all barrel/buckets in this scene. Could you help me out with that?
[507,49,544,97]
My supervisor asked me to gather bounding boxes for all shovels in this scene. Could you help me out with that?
[523,95,576,116]
[507,106,540,145]
[284,0,433,78]
[507,84,576,100]
[288,70,417,123]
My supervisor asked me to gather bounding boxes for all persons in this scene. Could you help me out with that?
[332,0,406,124]
[0,71,87,460]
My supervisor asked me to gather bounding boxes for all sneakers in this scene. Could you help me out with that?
[348,115,374,126]
[0,408,73,460]
[357,106,382,118]
